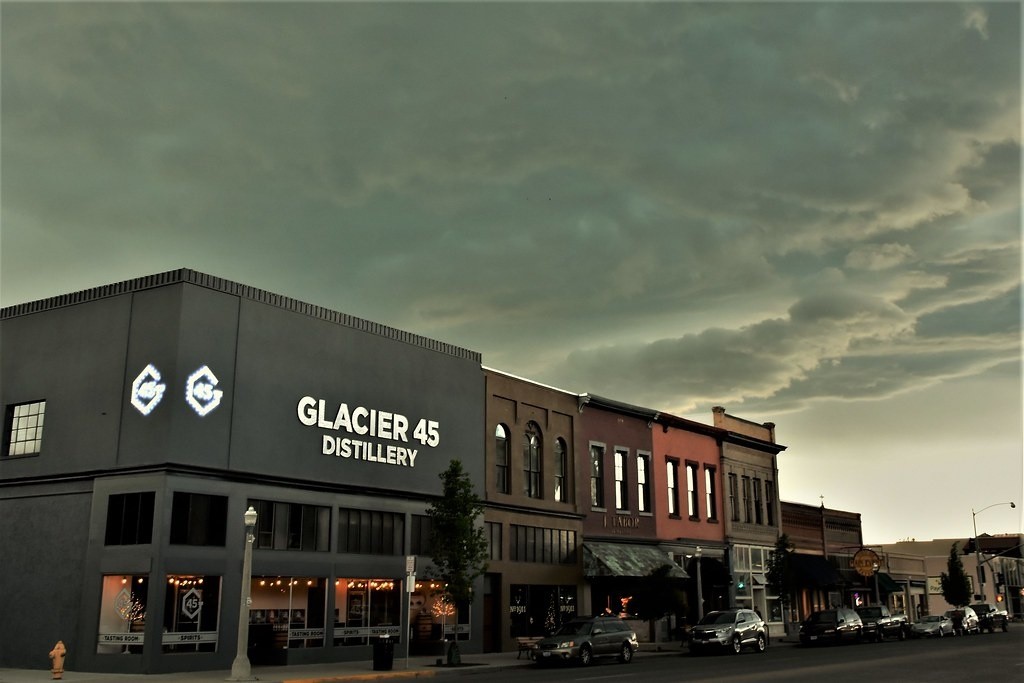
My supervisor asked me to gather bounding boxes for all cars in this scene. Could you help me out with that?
[798,609,864,643]
[909,615,956,637]
[531,617,640,666]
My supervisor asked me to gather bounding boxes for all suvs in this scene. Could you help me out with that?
[944,606,981,637]
[969,604,1009,635]
[690,609,767,654]
[855,605,911,643]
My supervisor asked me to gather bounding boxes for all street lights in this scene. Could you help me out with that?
[972,502,1017,600]
[872,563,882,607]
[230,505,259,680]
[695,546,705,618]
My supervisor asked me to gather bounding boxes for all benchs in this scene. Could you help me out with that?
[515,635,546,662]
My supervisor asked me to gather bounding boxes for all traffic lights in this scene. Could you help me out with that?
[997,596,1003,603]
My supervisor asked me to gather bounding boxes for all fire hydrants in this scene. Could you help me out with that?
[49,640,67,679]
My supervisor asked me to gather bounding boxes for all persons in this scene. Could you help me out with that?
[679,605,699,647]
[917,604,922,621]
[754,606,763,621]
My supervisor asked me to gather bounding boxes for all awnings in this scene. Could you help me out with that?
[870,573,903,594]
[582,541,690,578]
[784,552,855,594]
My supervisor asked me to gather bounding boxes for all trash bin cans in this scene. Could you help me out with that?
[373,638,395,671]
